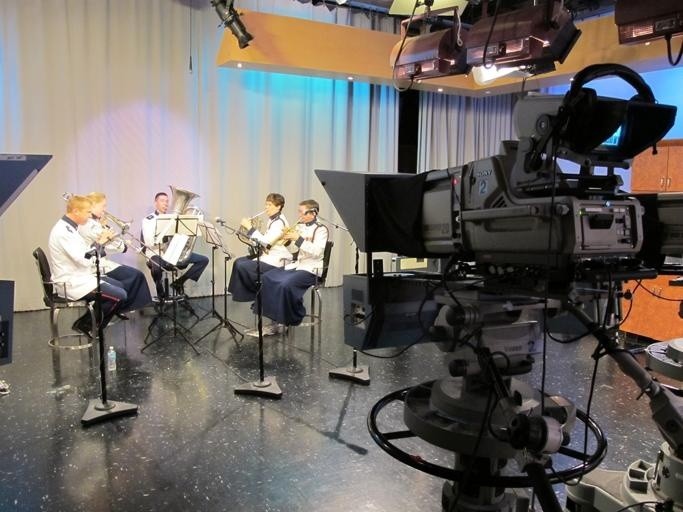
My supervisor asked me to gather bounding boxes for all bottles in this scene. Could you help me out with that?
[107,346,116,371]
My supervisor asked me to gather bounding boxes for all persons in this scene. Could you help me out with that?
[85,191,151,320]
[251,199,330,327]
[141,192,209,301]
[48,192,127,342]
[228,193,290,302]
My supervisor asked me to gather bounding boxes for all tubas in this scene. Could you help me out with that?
[157,185,205,266]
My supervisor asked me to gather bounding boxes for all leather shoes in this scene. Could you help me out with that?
[71,318,101,341]
[116,314,130,321]
[170,279,190,301]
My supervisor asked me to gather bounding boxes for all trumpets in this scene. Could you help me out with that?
[261,218,302,255]
[237,210,265,256]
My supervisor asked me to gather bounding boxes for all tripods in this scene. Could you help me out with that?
[186,247,237,335]
[193,256,244,352]
[141,264,202,355]
[163,235,200,321]
[144,244,194,344]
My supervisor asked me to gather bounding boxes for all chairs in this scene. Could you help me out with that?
[254,239,292,329]
[139,228,187,304]
[32,247,99,351]
[279,239,335,343]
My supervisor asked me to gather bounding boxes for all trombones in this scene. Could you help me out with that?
[63,191,182,280]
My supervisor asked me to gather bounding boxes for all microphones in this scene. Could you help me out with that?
[215,217,226,223]
[122,222,131,234]
[303,208,317,215]
